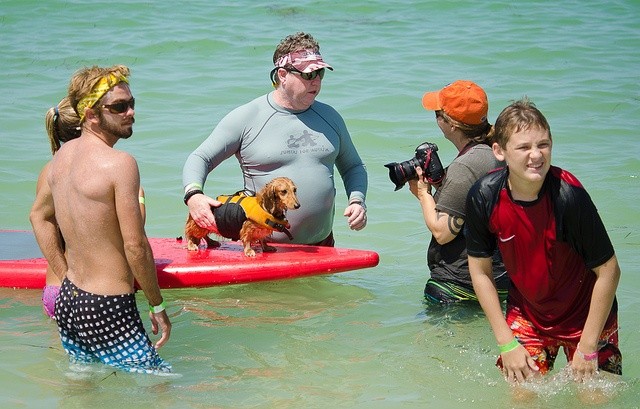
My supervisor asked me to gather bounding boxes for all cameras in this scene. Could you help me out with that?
[384,142,445,192]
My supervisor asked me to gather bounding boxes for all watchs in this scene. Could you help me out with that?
[349,201,367,213]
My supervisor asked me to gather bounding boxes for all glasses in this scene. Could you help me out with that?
[96,97,136,114]
[277,67,325,81]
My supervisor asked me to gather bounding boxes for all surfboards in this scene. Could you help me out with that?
[0,236,380,289]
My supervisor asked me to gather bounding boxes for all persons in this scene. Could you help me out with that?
[183,32,368,248]
[36,94,146,321]
[29,65,175,375]
[408,79,510,305]
[465,93,623,383]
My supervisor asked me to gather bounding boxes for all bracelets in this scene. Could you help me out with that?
[496,338,520,354]
[577,342,598,361]
[148,297,165,314]
[139,197,145,204]
[183,189,203,206]
[184,183,201,193]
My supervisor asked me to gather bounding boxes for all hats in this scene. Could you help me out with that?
[274,47,333,74]
[422,81,488,125]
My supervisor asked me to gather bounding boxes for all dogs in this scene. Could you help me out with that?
[185,177,301,257]
